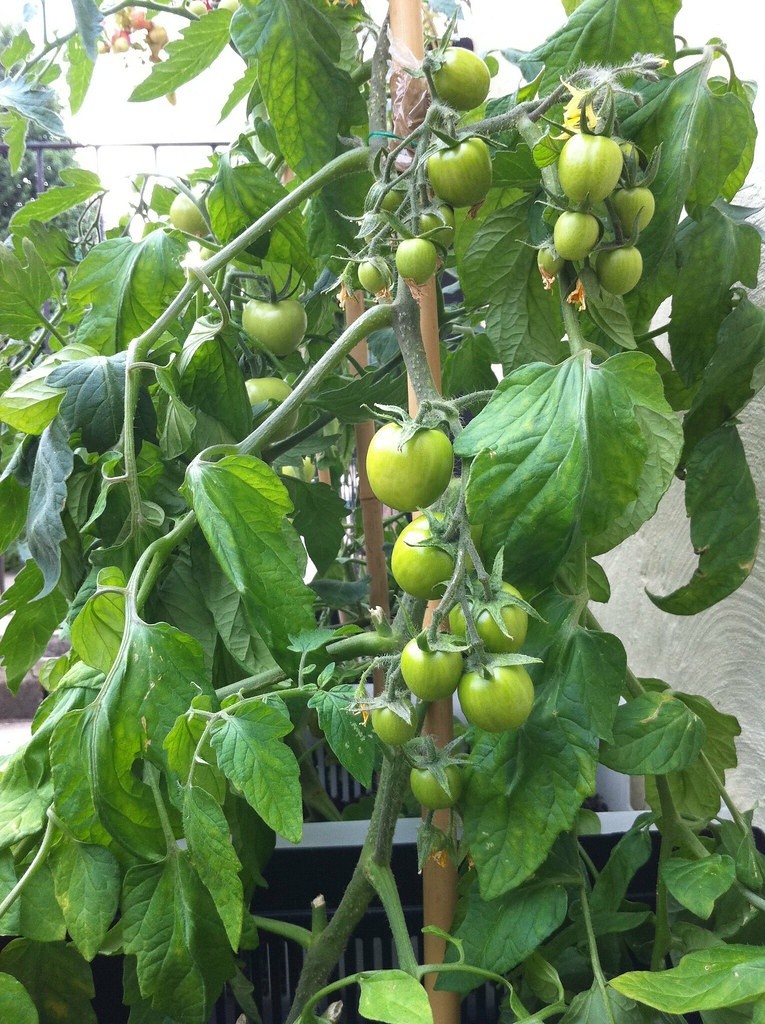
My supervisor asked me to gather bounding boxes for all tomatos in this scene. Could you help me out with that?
[98,0,656,810]
